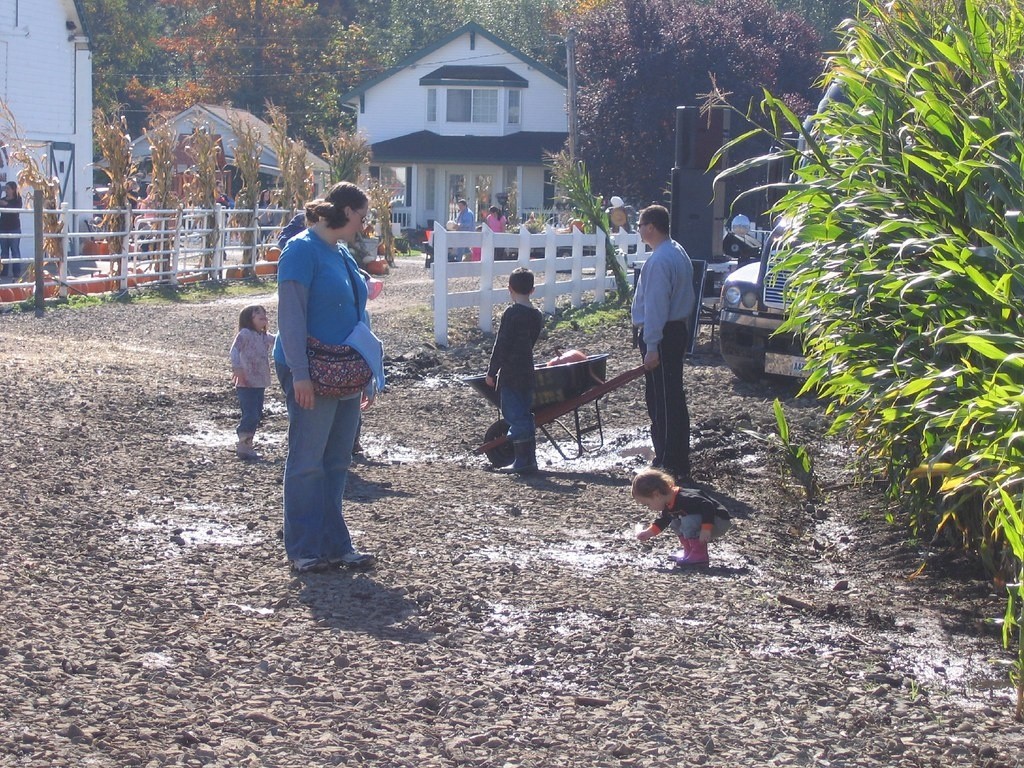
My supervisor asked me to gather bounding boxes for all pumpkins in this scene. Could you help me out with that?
[547,346,587,367]
[570,219,583,230]
[0,245,281,303]
[367,261,383,274]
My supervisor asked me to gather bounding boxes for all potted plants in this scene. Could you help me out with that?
[82,217,112,261]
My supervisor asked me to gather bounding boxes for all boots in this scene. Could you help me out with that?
[667,535,690,561]
[237,432,263,460]
[500,438,537,473]
[677,538,709,565]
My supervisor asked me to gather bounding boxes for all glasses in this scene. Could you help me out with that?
[636,221,649,230]
[352,208,368,223]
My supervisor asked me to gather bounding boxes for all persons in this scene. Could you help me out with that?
[272,181,375,575]
[486,268,543,474]
[632,204,696,475]
[0,181,23,283]
[92,176,507,272]
[277,198,371,457]
[631,468,728,565]
[229,304,277,460]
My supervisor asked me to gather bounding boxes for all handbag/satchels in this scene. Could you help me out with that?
[305,336,373,398]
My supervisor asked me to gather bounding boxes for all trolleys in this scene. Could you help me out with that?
[460,350,659,468]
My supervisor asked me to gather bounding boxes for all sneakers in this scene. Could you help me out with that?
[328,549,376,568]
[289,554,329,572]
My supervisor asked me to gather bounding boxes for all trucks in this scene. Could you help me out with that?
[717,57,887,386]
